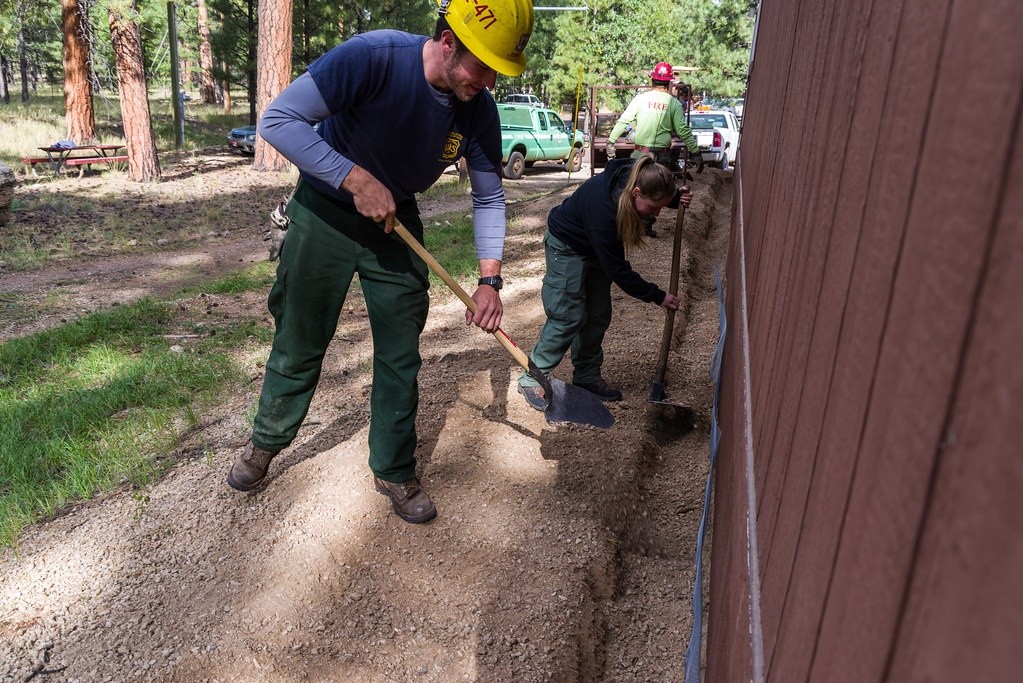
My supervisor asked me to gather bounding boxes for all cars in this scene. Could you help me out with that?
[227,126,255,158]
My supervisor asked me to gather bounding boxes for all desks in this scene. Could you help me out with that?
[38,143,125,179]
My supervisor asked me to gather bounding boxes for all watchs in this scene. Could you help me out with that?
[606,140,614,145]
[478,275,504,292]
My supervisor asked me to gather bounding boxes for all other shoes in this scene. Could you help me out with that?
[517,382,547,411]
[675,172,684,180]
[572,379,622,401]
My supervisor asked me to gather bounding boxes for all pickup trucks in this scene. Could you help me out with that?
[680,97,745,171]
[456,105,590,180]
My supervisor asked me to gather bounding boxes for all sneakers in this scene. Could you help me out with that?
[227,441,282,492]
[374,475,437,524]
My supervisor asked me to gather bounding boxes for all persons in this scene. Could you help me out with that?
[226,0,536,525]
[606,60,705,238]
[518,156,694,411]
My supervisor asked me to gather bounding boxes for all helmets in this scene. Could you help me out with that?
[437,0,534,77]
[649,61,675,80]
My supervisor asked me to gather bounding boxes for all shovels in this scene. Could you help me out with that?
[388,214,616,429]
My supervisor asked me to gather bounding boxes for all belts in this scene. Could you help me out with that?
[635,145,670,153]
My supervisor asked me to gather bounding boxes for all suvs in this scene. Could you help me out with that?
[506,94,544,108]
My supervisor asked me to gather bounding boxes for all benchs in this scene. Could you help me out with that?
[21,155,100,177]
[65,156,129,180]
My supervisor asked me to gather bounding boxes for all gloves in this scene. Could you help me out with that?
[693,152,704,175]
[606,140,616,159]
[260,201,291,261]
